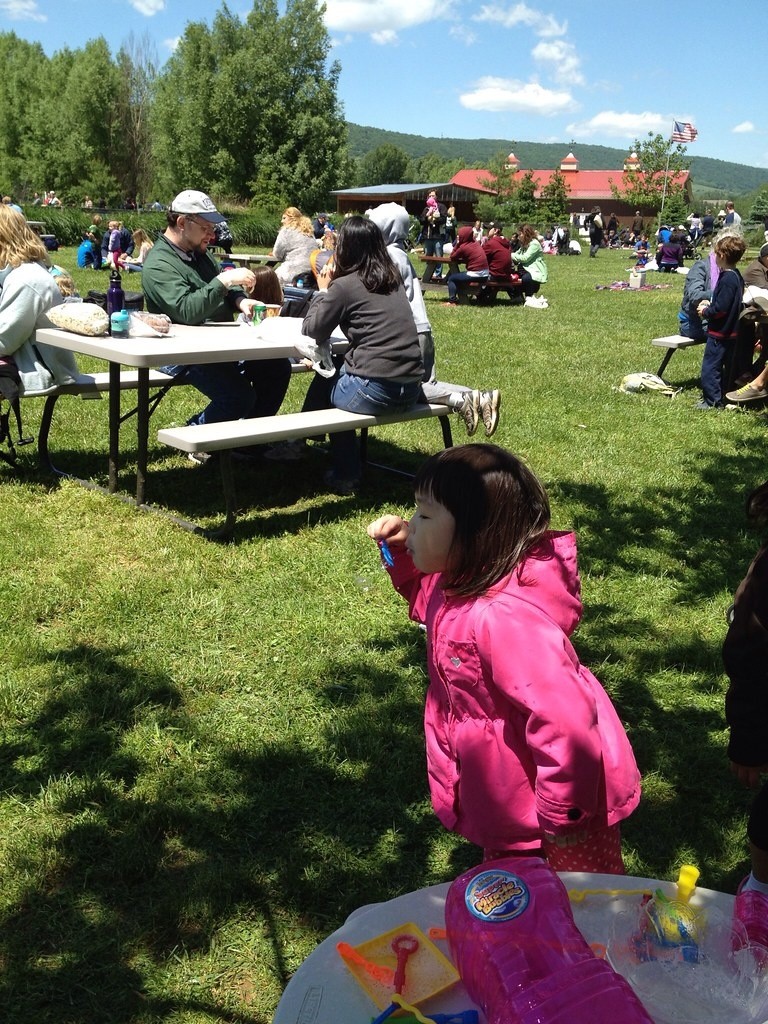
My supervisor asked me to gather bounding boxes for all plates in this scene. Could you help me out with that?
[204,321,240,326]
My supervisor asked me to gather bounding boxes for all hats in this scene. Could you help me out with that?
[759,242,768,257]
[168,190,228,223]
[483,221,503,231]
[319,214,329,220]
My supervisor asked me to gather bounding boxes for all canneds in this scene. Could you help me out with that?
[252,304,267,326]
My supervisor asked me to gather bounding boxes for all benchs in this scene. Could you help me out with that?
[653,333,708,376]
[417,274,524,305]
[39,234,55,240]
[38,363,454,544]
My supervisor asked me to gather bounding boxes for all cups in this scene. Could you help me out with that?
[265,303,281,318]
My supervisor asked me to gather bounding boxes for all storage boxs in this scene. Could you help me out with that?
[630,272,646,288]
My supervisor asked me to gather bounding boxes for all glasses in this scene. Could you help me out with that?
[185,217,216,233]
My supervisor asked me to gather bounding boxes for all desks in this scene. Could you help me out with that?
[25,220,46,236]
[212,252,283,272]
[35,316,352,505]
[418,255,526,305]
[271,870,768,1024]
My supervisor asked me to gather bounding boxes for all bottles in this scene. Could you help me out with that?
[110,309,129,339]
[107,270,125,316]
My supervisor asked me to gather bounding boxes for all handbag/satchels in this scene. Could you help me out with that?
[593,213,603,228]
[0,355,34,446]
[621,373,684,399]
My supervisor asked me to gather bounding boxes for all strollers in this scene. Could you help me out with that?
[682,225,704,262]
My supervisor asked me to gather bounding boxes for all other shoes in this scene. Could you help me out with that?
[188,450,212,464]
[233,445,273,459]
[511,295,523,303]
[125,266,129,275]
[698,402,724,410]
[439,302,457,307]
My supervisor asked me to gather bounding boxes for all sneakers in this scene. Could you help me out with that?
[264,439,309,461]
[323,471,359,492]
[453,389,480,437]
[479,389,501,438]
[726,383,768,402]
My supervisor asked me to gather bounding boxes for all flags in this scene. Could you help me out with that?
[673,121,698,143]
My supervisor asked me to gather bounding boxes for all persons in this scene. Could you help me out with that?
[151,199,162,211]
[584,206,606,258]
[273,207,329,285]
[365,206,373,215]
[33,191,62,206]
[84,196,92,208]
[0,203,70,377]
[2,196,22,213]
[124,197,137,210]
[144,190,292,467]
[367,444,642,875]
[99,197,106,208]
[369,202,501,438]
[680,225,768,410]
[573,212,578,225]
[606,211,653,266]
[78,215,152,273]
[534,230,581,256]
[265,216,425,488]
[209,211,234,255]
[687,201,741,250]
[720,543,768,899]
[420,189,548,307]
[344,210,351,217]
[658,225,690,274]
